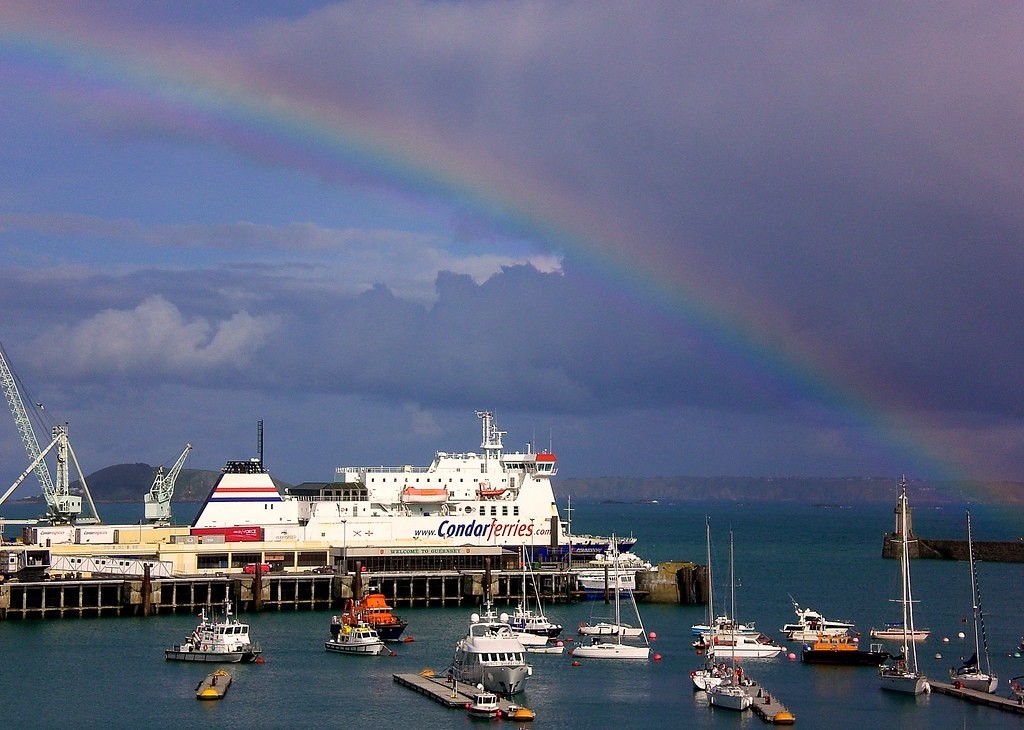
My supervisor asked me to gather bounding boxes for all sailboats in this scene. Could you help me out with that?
[703,530,753,712]
[867,474,932,642]
[687,513,731,692]
[876,490,932,698]
[570,540,650,660]
[577,534,644,640]
[950,507,998,693]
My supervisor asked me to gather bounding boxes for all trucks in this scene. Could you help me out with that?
[243,563,269,574]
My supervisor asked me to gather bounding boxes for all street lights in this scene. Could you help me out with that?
[340,519,348,575]
[529,518,536,563]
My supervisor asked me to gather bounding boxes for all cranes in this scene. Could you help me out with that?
[141,444,193,531]
[0,348,102,527]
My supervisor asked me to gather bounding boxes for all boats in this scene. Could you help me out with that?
[446,588,535,697]
[464,690,502,720]
[691,593,891,668]
[164,596,267,665]
[189,408,639,573]
[339,589,410,645]
[400,486,449,507]
[324,620,386,658]
[479,482,506,497]
[499,541,565,657]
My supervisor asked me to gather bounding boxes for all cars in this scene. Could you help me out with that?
[312,564,338,574]
